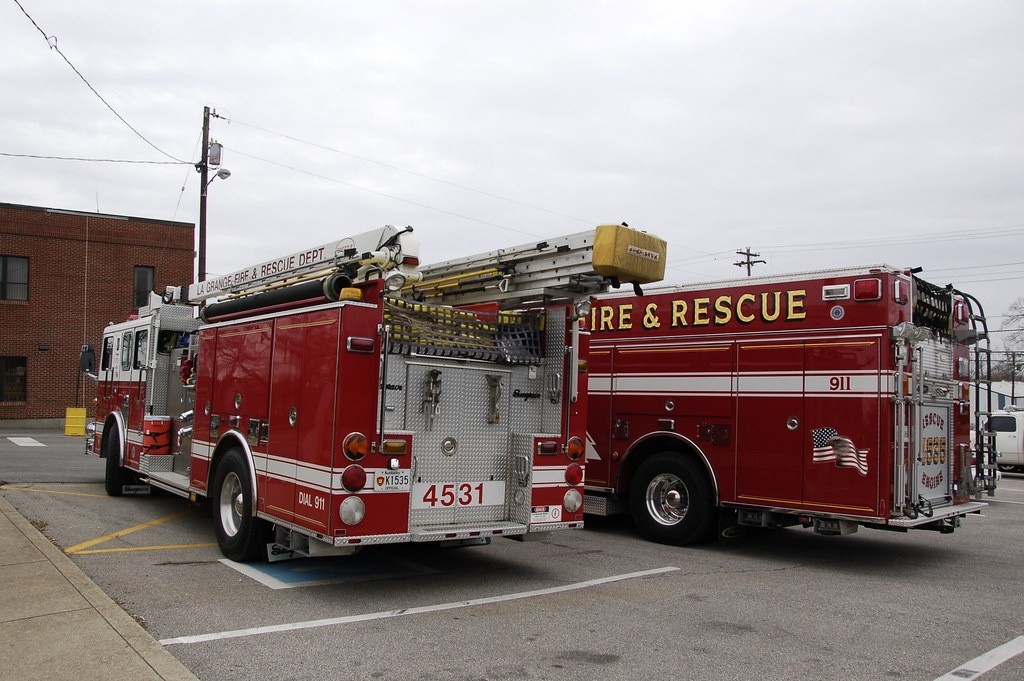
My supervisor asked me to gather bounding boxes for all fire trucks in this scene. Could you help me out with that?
[575,262,1005,548]
[80,223,669,564]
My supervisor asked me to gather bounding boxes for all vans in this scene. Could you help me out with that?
[968,407,1024,479]
